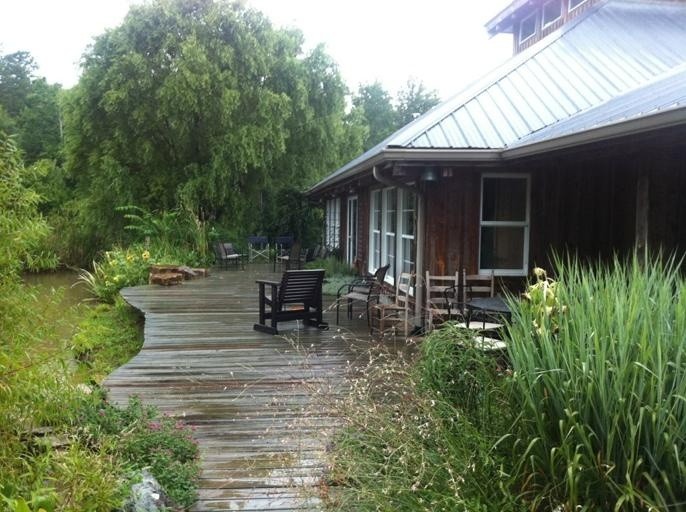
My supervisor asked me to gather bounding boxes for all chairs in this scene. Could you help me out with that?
[210,240,329,335]
[337,264,494,340]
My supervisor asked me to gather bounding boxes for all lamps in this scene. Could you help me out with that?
[442,166,452,178]
[420,167,438,183]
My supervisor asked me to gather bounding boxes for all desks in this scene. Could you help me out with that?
[469,298,511,324]
